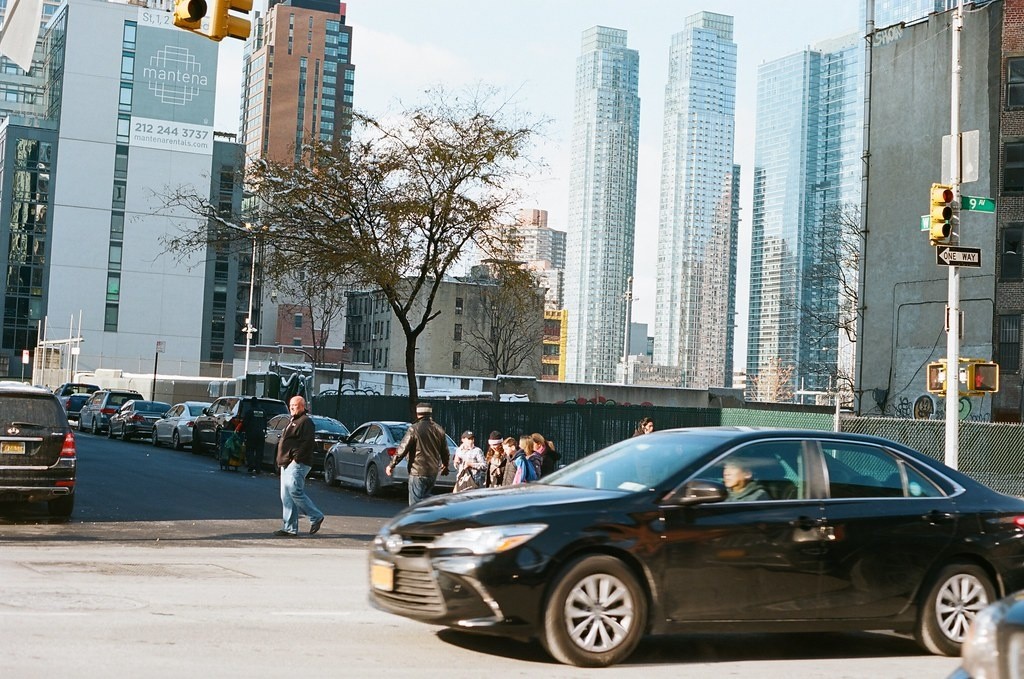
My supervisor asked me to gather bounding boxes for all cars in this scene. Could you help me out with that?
[108,399,173,441]
[242,414,350,479]
[323,422,459,497]
[151,401,213,449]
[368,426,1024,679]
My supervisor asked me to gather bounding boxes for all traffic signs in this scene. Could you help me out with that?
[936,245,982,268]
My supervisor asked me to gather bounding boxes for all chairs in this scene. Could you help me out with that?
[758,455,799,501]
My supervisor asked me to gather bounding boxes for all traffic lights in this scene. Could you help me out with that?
[929,183,954,247]
[926,362,947,394]
[967,363,999,393]
[209,0,254,42]
[174,0,207,30]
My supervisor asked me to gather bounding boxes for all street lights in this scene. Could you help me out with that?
[242,220,268,379]
[294,348,315,397]
[623,276,634,385]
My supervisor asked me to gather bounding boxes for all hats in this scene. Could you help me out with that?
[461,430,475,438]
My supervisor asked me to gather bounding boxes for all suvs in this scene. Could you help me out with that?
[78,388,145,435]
[192,395,290,460]
[0,381,77,516]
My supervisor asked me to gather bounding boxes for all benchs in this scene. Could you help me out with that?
[827,473,903,498]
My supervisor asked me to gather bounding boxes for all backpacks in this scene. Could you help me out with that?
[512,455,537,482]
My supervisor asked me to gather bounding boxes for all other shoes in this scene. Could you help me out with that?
[248,464,255,472]
[273,530,293,536]
[310,517,324,533]
[256,468,260,472]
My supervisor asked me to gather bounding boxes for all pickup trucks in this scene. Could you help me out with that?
[54,383,101,422]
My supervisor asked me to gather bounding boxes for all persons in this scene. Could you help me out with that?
[723,454,771,502]
[486,431,543,488]
[451,430,488,493]
[386,403,449,508]
[234,397,268,480]
[274,396,324,536]
[532,433,562,478]
[633,417,654,436]
